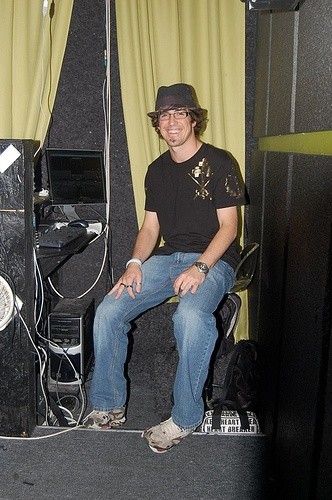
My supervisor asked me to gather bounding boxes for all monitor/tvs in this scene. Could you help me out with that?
[45,147,107,222]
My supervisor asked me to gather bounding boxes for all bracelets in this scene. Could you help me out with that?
[125,258,142,268]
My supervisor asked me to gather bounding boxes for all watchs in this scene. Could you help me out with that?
[193,262,209,275]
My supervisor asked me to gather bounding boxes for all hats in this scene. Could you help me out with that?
[146,83,208,119]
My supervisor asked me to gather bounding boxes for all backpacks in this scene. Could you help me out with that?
[211,340,264,430]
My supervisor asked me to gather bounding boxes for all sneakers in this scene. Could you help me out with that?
[140,416,197,453]
[80,410,127,430]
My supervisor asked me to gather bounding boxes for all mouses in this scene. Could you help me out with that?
[68,220,89,228]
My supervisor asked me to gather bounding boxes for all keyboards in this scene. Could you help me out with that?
[38,228,87,248]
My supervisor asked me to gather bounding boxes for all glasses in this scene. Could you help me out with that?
[158,112,192,121]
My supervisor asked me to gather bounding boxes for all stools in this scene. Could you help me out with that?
[198,242,260,409]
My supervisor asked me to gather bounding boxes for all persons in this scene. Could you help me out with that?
[80,83,249,452]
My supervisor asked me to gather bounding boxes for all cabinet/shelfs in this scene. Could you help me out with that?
[0,138,100,438]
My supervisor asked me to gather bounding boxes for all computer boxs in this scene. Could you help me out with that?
[48,297,96,394]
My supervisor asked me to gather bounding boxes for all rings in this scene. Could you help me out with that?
[126,285,133,288]
[135,283,142,286]
[121,283,126,287]
